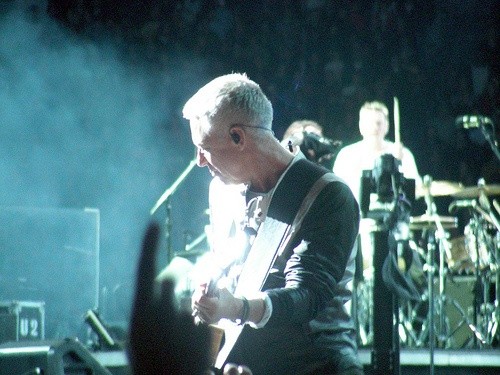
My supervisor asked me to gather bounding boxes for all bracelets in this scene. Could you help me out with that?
[231,295,249,325]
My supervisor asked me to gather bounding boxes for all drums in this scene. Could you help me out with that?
[444,236,475,273]
[463,221,494,266]
[406,240,434,345]
[427,279,477,349]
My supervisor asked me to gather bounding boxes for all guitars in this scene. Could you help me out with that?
[190,248,238,375]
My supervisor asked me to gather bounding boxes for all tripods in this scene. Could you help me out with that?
[396,202,500,350]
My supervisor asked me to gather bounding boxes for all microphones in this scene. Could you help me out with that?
[455,115,491,129]
[424,175,433,202]
[479,179,489,202]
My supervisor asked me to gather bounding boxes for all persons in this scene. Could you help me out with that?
[182,70,364,375]
[333,101,427,343]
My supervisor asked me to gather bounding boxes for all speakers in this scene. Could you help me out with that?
[0,338,113,375]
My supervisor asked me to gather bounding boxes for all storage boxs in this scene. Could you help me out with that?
[434,277,497,351]
[0,300,45,343]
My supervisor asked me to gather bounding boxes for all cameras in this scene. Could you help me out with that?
[301,132,342,161]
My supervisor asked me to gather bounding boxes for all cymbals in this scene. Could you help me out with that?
[452,184,500,197]
[410,216,458,229]
[429,181,463,196]
